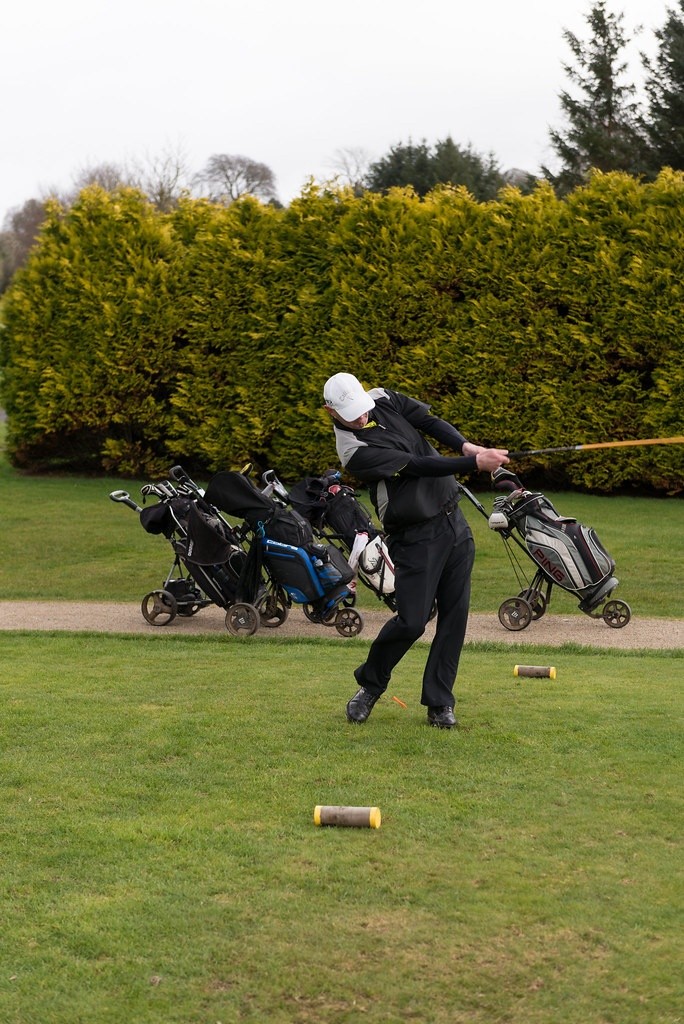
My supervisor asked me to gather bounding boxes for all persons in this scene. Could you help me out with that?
[323,372,511,731]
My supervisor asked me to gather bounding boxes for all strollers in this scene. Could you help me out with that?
[456,467,631,631]
[109,465,440,637]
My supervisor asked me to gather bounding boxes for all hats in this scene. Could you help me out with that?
[323,372,375,422]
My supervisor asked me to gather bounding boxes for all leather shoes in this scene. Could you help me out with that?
[428,705,458,729]
[346,687,380,723]
